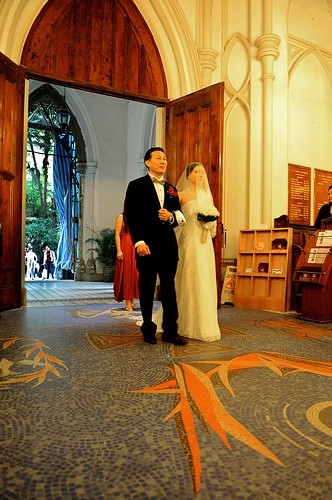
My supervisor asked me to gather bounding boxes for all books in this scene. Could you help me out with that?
[307,230,332,263]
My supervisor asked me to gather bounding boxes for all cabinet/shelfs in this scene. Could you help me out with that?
[233,228,293,313]
[291,229,332,324]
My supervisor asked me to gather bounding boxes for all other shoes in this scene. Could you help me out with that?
[126,303,139,311]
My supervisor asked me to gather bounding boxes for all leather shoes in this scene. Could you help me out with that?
[162,337,188,346]
[144,337,158,344]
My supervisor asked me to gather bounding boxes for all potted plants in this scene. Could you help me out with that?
[84,225,117,282]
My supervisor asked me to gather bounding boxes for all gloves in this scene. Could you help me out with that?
[204,222,217,230]
[174,210,187,225]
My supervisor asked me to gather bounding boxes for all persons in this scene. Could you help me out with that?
[135,162,221,342]
[122,147,188,346]
[314,185,332,229]
[112,210,140,311]
[24,242,57,279]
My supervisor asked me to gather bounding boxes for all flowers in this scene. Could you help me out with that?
[198,205,220,242]
[167,186,178,198]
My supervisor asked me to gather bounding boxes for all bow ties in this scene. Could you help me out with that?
[152,176,165,185]
